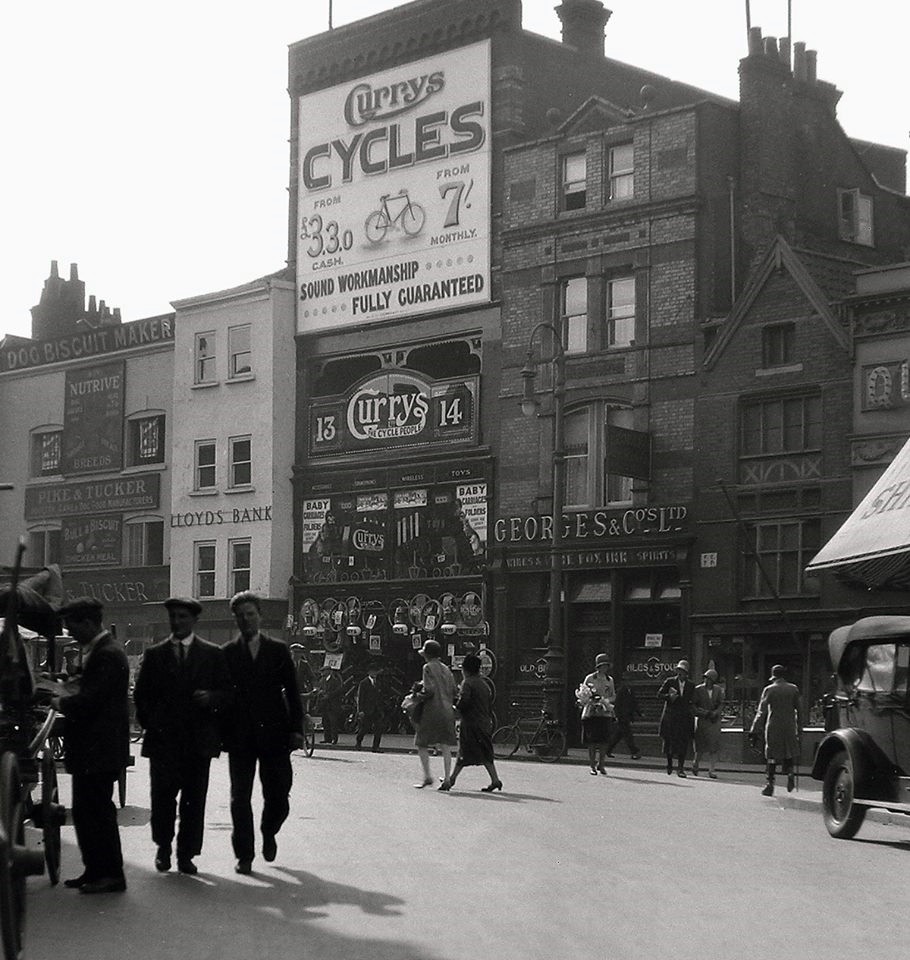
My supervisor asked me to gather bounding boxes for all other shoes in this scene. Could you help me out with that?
[438,782,452,792]
[320,740,332,743]
[79,880,127,894]
[590,769,597,775]
[692,765,698,776]
[481,780,502,792]
[413,782,433,789]
[64,873,89,888]
[177,859,197,874]
[597,766,606,774]
[667,765,673,775]
[262,836,277,862]
[709,772,717,778]
[332,740,337,744]
[356,743,362,751]
[631,755,641,760]
[677,767,686,778]
[371,746,385,753]
[155,847,172,871]
[606,754,615,758]
[235,861,251,874]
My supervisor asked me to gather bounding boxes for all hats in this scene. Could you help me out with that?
[677,660,689,673]
[164,597,203,615]
[57,595,105,615]
[418,640,442,658]
[595,653,610,668]
[462,654,482,670]
[702,669,718,683]
[769,665,787,680]
[622,671,632,679]
[320,666,331,671]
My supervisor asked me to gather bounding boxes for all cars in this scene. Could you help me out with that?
[809,615,909,841]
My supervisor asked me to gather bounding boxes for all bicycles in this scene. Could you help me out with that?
[491,702,567,763]
[295,673,453,759]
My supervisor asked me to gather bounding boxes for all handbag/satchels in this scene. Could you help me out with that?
[401,697,425,723]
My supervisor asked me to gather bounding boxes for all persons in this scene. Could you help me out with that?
[656,659,695,778]
[318,666,342,745]
[577,654,644,775]
[356,662,386,753]
[132,595,223,874]
[50,595,131,895]
[408,639,457,791]
[289,643,321,694]
[747,665,805,797]
[690,669,725,778]
[219,589,305,874]
[438,654,503,792]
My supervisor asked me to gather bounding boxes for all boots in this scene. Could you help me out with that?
[762,780,774,796]
[787,774,795,792]
